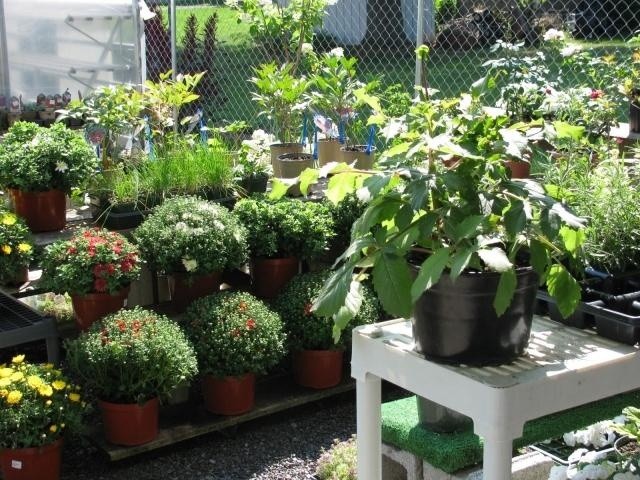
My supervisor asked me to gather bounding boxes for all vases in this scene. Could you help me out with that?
[167,272,223,307]
[69,291,131,327]
[1,437,66,479]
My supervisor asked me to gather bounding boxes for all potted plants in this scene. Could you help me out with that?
[246,61,311,165]
[339,71,408,168]
[130,193,251,267]
[310,112,584,367]
[223,119,269,196]
[539,151,640,308]
[306,46,356,167]
[101,90,142,155]
[174,292,292,416]
[265,109,317,194]
[228,197,337,301]
[80,78,239,224]
[0,213,34,288]
[468,40,570,178]
[277,268,381,388]
[0,119,99,234]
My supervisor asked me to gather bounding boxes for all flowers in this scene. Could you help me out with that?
[192,218,196,219]
[63,305,194,446]
[1,354,100,445]
[39,224,144,290]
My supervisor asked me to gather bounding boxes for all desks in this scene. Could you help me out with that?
[348,316,639,480]
